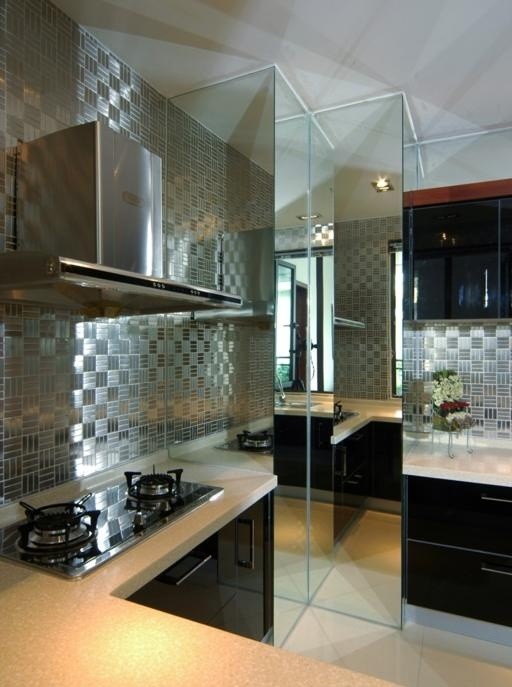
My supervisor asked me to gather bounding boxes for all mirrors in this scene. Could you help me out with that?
[165,66,421,643]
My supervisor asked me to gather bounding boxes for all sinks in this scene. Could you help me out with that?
[274,402,324,409]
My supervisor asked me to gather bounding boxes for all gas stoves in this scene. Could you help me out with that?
[0,464,225,580]
[213,423,274,457]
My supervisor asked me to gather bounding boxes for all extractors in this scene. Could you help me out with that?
[192,225,368,329]
[1,119,244,316]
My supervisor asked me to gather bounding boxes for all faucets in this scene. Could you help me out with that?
[275,375,285,402]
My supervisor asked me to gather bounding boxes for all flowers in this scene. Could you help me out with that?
[434,369,474,432]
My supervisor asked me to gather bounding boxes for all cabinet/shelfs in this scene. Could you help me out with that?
[412,197,511,323]
[403,473,512,667]
[124,489,273,652]
[275,415,403,516]
